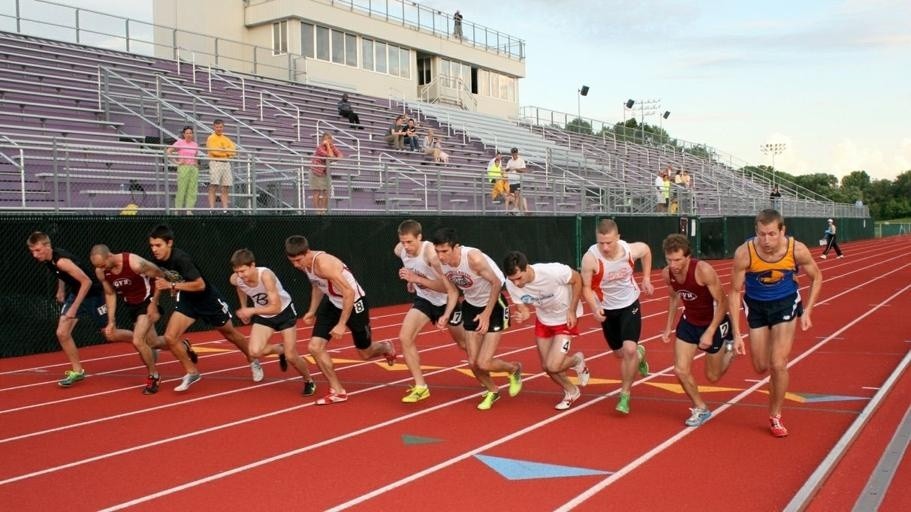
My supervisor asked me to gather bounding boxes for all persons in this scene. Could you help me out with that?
[150,223,264,392]
[728,184,845,438]
[454,10,463,43]
[89,243,198,395]
[309,132,344,215]
[581,219,656,415]
[27,231,158,388]
[166,125,199,216]
[503,251,591,410]
[399,220,486,403]
[487,148,530,215]
[654,163,691,214]
[206,119,237,214]
[284,235,397,406]
[661,234,734,427]
[229,248,317,396]
[338,94,364,130]
[433,227,522,410]
[384,110,448,167]
[856,198,863,217]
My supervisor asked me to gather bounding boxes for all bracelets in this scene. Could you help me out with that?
[171,282,176,290]
